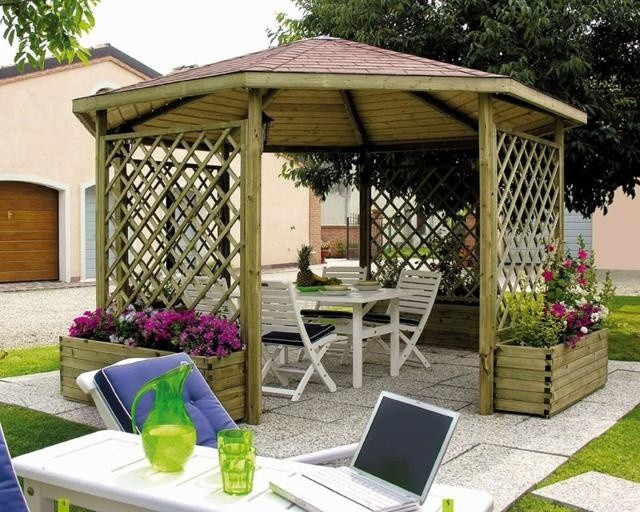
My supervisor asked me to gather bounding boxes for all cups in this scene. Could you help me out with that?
[216,427,254,473]
[218,441,255,496]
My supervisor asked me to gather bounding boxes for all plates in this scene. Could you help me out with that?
[296,285,323,292]
[351,280,381,291]
[319,284,352,296]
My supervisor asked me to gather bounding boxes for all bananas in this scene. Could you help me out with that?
[312,272,328,282]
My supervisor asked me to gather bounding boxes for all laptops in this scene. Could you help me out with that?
[269,391,461,512]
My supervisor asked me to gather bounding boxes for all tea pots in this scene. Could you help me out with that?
[131,363,199,472]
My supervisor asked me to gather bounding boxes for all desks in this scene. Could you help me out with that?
[10,430,493,512]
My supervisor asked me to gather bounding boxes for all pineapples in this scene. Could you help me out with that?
[296,243,314,287]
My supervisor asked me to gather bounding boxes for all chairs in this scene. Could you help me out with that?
[178,265,443,402]
[75,352,245,449]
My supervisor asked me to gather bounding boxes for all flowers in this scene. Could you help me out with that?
[66,304,242,363]
[503,234,618,350]
